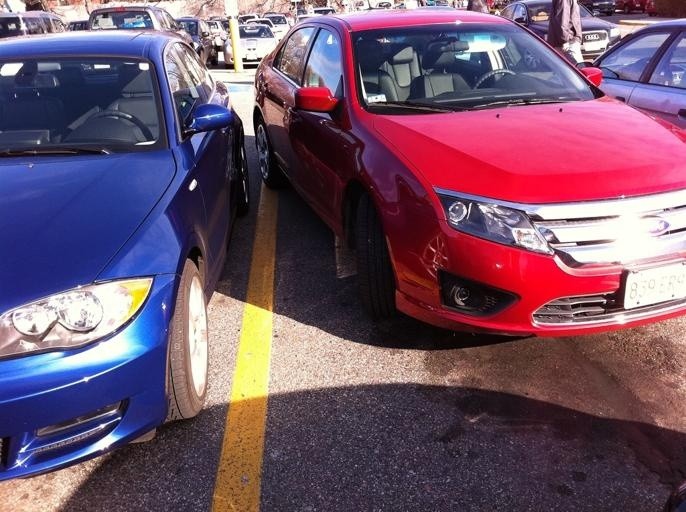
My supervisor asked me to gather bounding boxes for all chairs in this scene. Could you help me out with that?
[1,76,66,137]
[352,36,400,107]
[380,36,422,102]
[408,46,471,100]
[109,64,161,142]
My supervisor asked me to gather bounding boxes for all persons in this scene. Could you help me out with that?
[469,0,488,14]
[549,0,585,64]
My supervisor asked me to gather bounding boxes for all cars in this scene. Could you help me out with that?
[547,18,686,132]
[0,27,250,482]
[253,9,686,338]
